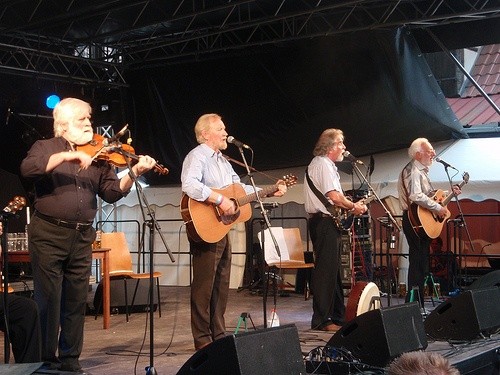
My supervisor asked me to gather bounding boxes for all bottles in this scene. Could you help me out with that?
[268,308,279,327]
[95,229,101,249]
[304,282,309,299]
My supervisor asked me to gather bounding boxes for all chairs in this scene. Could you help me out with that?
[95,232,163,323]
[263,228,315,304]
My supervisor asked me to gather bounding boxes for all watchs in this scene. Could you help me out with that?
[214,195,225,205]
[348,206,355,212]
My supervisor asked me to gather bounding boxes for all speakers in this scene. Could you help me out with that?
[470,270,500,289]
[322,300,428,370]
[175,324,307,375]
[423,286,500,340]
[109,277,156,313]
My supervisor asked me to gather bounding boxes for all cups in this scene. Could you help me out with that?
[400,281,406,296]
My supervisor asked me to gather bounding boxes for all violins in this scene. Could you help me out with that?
[76,132,169,174]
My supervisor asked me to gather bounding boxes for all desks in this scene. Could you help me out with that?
[0,247,113,330]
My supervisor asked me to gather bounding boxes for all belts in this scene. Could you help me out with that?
[33,209,94,232]
[309,213,331,218]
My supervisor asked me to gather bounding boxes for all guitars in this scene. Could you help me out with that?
[0,195,26,227]
[332,194,375,231]
[408,171,470,238]
[179,173,298,245]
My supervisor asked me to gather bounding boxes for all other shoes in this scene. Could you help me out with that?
[321,323,343,330]
[71,368,90,375]
[420,307,432,315]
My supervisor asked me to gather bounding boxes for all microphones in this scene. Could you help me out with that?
[102,128,129,146]
[343,151,364,165]
[436,156,454,169]
[226,136,250,149]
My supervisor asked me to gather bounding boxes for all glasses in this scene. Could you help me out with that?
[420,149,436,154]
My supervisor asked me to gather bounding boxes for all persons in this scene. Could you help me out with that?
[180,113,287,350]
[0,220,42,363]
[22,95,155,374]
[385,350,460,375]
[398,137,462,318]
[303,129,368,337]
[429,238,457,292]
